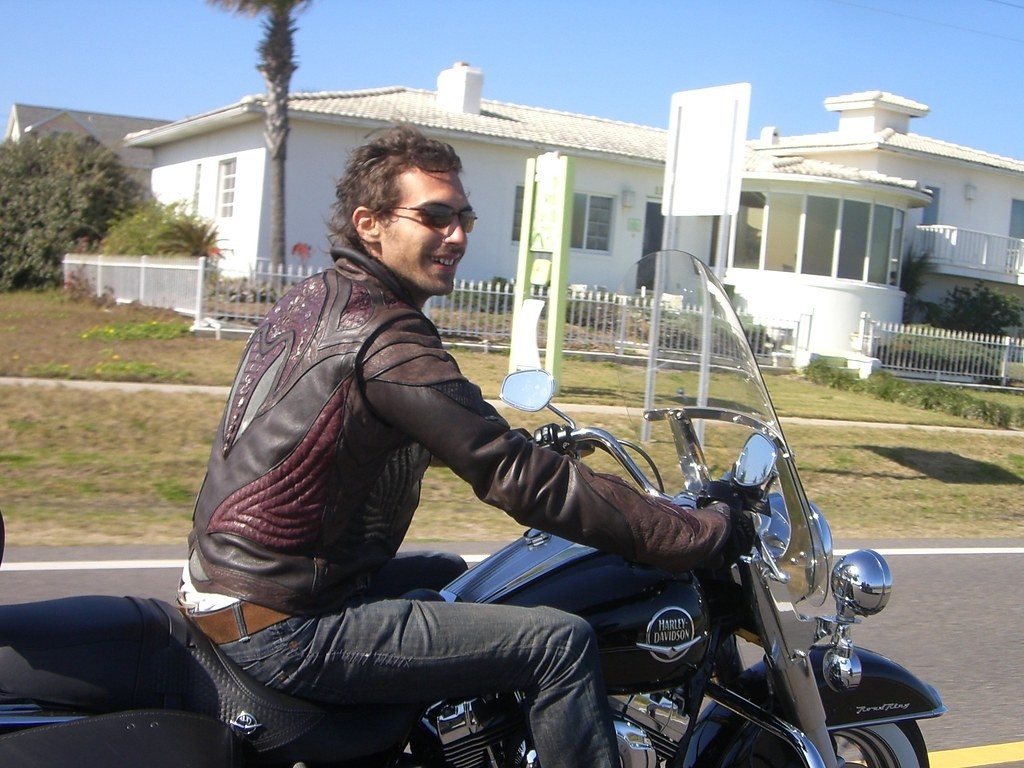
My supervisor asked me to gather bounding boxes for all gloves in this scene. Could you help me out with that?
[723,506,756,562]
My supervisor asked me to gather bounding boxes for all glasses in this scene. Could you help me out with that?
[389,203,478,233]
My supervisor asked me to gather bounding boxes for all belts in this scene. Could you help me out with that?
[175,600,295,646]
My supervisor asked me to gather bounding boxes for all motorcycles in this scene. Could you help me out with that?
[0,247,945,768]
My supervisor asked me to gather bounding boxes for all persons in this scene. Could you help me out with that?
[174,123,754,768]
